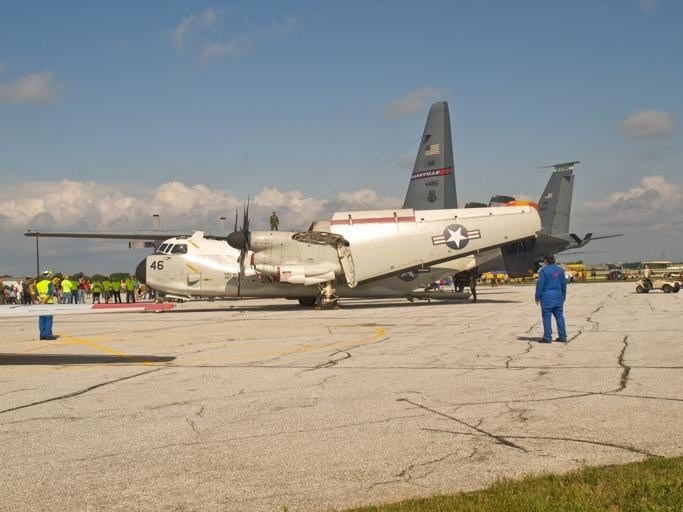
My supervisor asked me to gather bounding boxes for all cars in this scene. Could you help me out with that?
[636,277,681,293]
[608,270,625,280]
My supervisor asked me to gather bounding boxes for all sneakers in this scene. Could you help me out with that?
[538,338,567,343]
[39,335,56,341]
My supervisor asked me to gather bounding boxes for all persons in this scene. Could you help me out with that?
[433,278,440,293]
[575,271,579,281]
[0,270,162,304]
[440,279,444,289]
[640,263,653,288]
[270,211,279,231]
[492,271,498,287]
[458,266,477,303]
[534,252,567,343]
[569,271,573,282]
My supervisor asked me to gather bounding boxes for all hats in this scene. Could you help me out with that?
[42,270,49,278]
